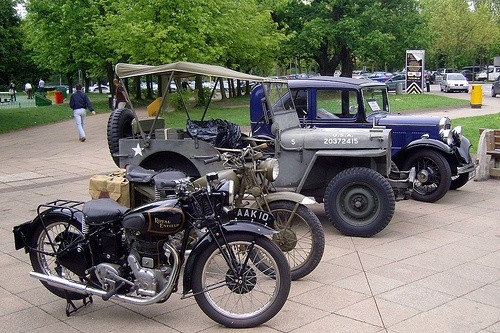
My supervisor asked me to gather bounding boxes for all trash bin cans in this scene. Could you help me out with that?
[55,91,63,104]
[395,82,403,95]
[470,84,483,108]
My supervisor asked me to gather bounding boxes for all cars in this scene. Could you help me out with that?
[435,67,476,94]
[353,70,435,92]
[88,79,248,93]
[491,75,500,97]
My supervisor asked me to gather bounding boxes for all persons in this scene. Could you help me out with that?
[36,78,45,93]
[424,72,431,92]
[286,74,291,80]
[182,79,189,91]
[24,81,33,99]
[69,84,95,142]
[298,72,302,80]
[9,80,16,101]
[113,79,129,109]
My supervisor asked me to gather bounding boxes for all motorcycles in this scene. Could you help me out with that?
[88,142,326,281]
[13,171,292,328]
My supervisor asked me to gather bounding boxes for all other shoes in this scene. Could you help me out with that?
[80,137,86,141]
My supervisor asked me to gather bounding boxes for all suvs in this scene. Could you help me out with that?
[107,60,427,237]
[249,75,480,204]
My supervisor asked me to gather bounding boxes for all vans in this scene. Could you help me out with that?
[475,66,500,80]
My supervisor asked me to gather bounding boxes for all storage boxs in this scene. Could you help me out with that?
[155,128,185,140]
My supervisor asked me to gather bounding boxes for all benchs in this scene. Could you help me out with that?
[479,128,500,177]
